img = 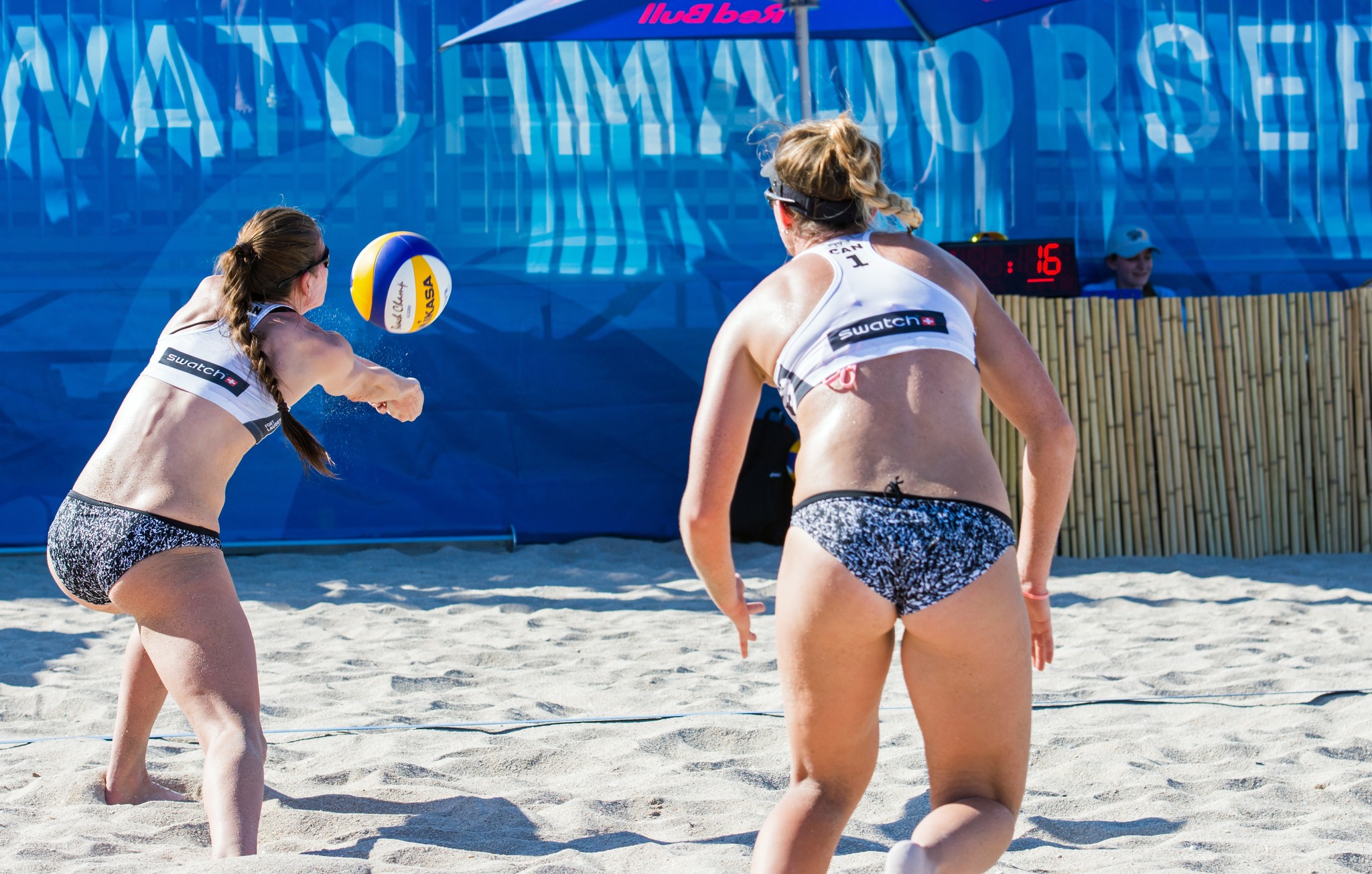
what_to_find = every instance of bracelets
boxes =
[1020,589,1051,603]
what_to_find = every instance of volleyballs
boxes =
[786,439,802,483]
[349,231,452,334]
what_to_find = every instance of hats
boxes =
[1107,225,1160,258]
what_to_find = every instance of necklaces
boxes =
[805,230,841,245]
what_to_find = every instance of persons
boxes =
[1080,224,1176,300]
[45,207,424,859]
[679,111,1077,874]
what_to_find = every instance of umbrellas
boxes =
[441,0,1060,121]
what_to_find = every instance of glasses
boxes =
[277,245,330,289]
[971,232,1008,242]
